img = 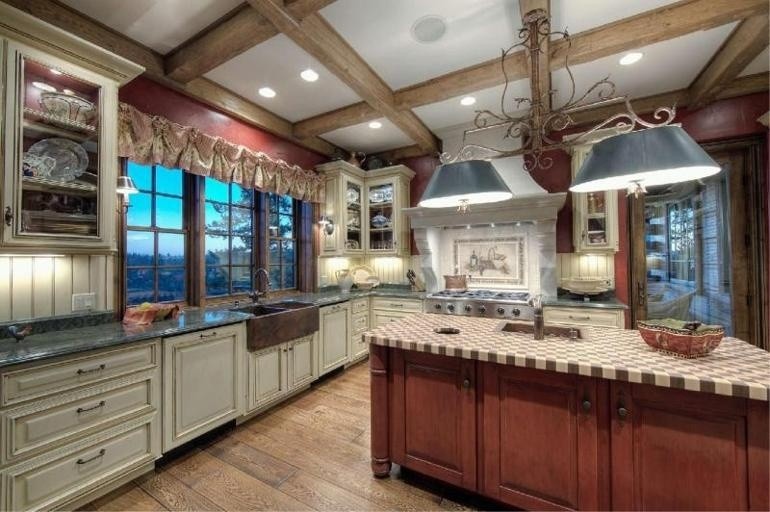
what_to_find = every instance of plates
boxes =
[28,137,90,183]
[353,265,381,290]
[346,186,393,227]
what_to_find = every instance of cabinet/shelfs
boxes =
[344,295,371,370]
[0,336,164,512]
[236,332,319,426]
[390,348,482,495]
[319,300,352,378]
[371,296,425,329]
[315,159,364,257]
[1,2,147,256]
[365,164,416,255]
[163,321,245,456]
[562,125,619,255]
[540,306,625,329]
[482,361,770,512]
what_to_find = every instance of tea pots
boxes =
[21,145,56,179]
[335,269,353,294]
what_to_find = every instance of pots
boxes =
[443,275,467,293]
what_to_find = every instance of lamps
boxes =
[116,176,139,215]
[319,215,334,235]
[417,9,723,214]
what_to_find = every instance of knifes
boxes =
[408,270,415,285]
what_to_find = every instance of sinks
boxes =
[266,302,317,310]
[228,306,287,319]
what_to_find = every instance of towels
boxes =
[122,301,180,326]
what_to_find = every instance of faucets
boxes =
[252,268,273,290]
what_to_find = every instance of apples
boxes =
[141,302,153,310]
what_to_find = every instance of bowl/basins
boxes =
[40,90,97,125]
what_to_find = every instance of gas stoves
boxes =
[423,290,531,318]
